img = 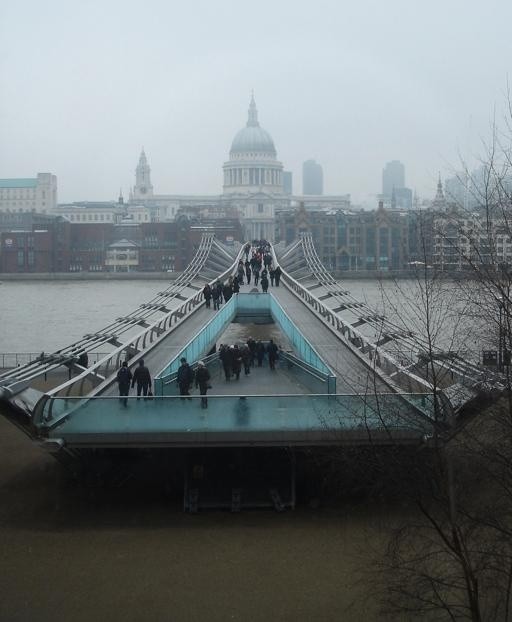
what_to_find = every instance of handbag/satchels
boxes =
[206,383,211,388]
[148,387,153,400]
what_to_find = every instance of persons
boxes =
[195,359,212,409]
[131,359,152,402]
[234,397,249,428]
[202,238,283,311]
[219,335,279,382]
[175,357,194,401]
[117,360,133,408]
